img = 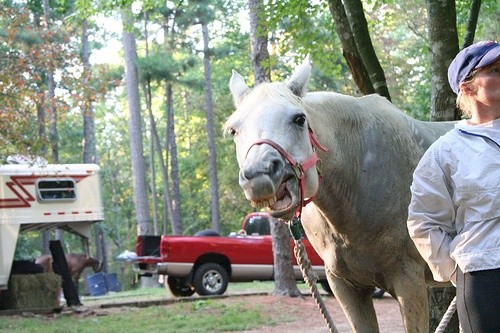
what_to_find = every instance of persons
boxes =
[407,40,500,333]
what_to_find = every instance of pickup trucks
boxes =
[135,212,386,298]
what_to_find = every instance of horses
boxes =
[220,52,468,333]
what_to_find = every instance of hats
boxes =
[448,41,500,95]
